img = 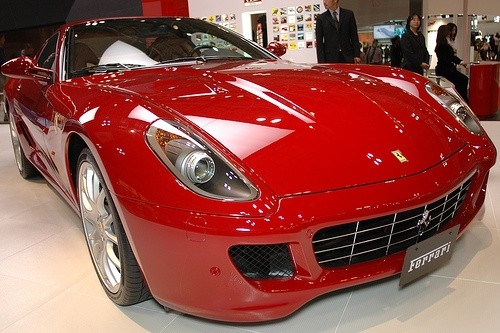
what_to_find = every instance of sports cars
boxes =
[0,15,497,324]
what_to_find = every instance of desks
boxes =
[469,61,500,121]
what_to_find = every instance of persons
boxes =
[0,32,7,65]
[435,25,469,104]
[364,35,400,66]
[313,0,362,65]
[471,31,500,62]
[398,13,430,74]
[446,23,458,69]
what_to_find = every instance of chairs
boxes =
[74,34,199,69]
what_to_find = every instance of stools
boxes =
[428,75,455,87]
